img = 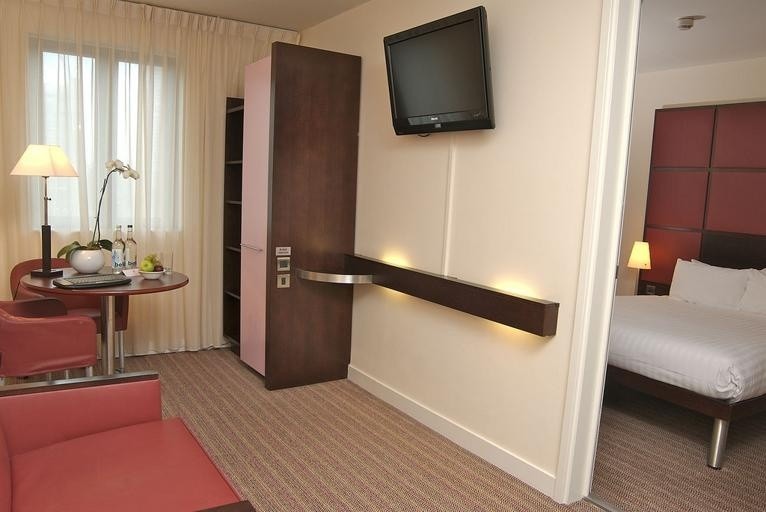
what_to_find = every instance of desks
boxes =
[20,266,190,373]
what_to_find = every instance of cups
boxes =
[160,246,174,276]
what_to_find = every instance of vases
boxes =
[68,245,105,275]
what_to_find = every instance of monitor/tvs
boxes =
[382,5,495,135]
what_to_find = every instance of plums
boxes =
[154,266,163,271]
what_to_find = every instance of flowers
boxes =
[57,158,141,263]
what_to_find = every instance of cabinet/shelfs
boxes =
[241,42,362,390]
[223,96,243,346]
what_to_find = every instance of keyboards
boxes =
[51,273,131,289]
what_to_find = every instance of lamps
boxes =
[9,144,81,277]
[627,240,653,295]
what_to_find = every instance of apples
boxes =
[141,260,154,272]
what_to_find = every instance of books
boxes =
[113,268,143,277]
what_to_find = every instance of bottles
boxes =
[125,224,137,268]
[111,224,126,274]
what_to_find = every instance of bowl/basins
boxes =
[138,269,165,280]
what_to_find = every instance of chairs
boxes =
[10,253,130,369]
[0,297,96,376]
[0,368,259,512]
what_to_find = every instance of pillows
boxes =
[669,258,750,310]
[760,265,765,273]
[691,257,754,271]
[738,268,766,314]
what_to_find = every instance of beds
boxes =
[605,228,766,470]
